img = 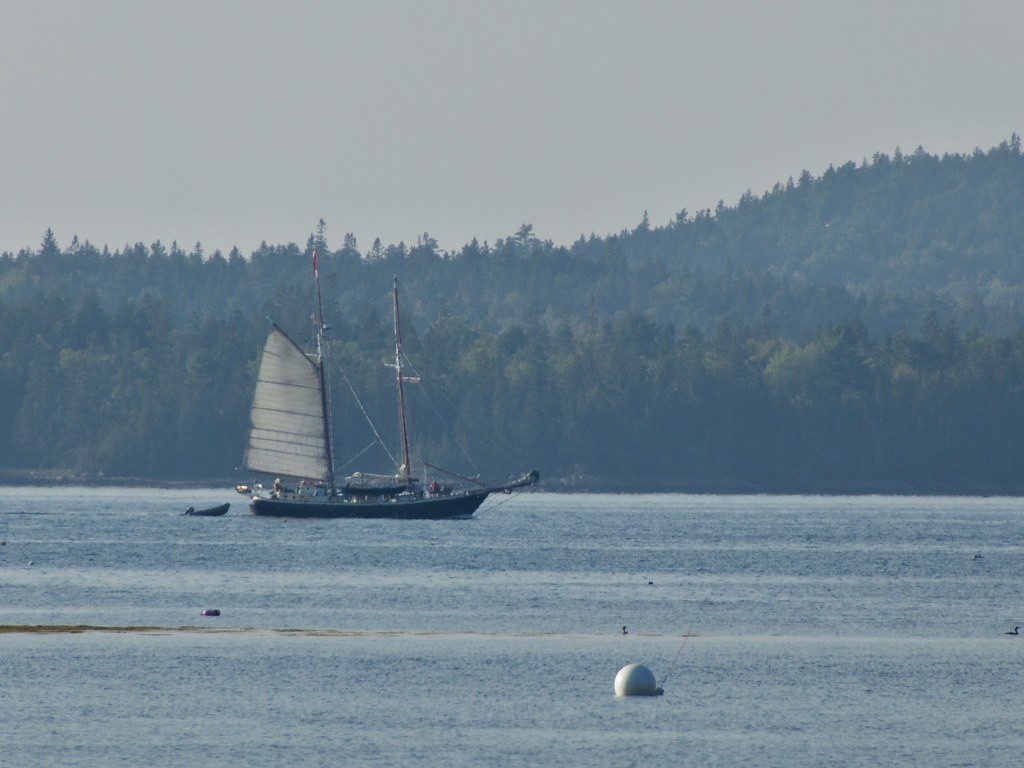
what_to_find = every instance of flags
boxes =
[312,250,317,278]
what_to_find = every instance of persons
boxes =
[274,477,280,498]
[432,481,437,498]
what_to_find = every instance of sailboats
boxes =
[235,249,539,519]
